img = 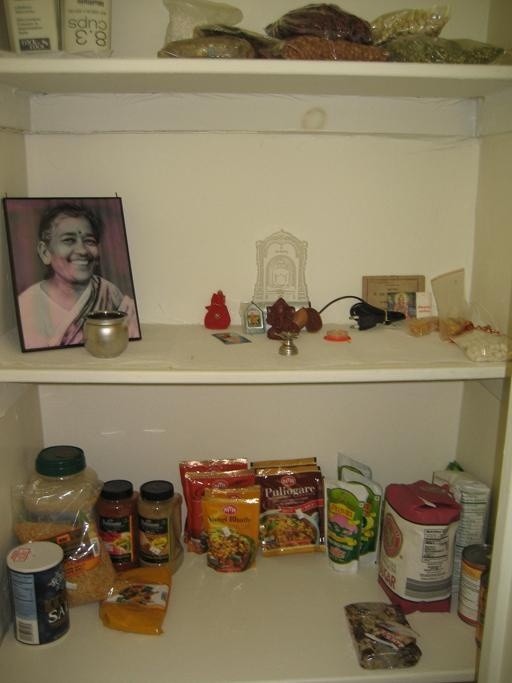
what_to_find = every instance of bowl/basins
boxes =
[83,312,131,360]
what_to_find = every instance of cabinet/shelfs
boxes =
[0,0,511,677]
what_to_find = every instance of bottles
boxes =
[377,481,463,614]
[474,569,489,647]
[136,478,186,574]
[95,478,139,571]
[456,542,492,625]
[5,539,72,646]
[22,444,100,522]
